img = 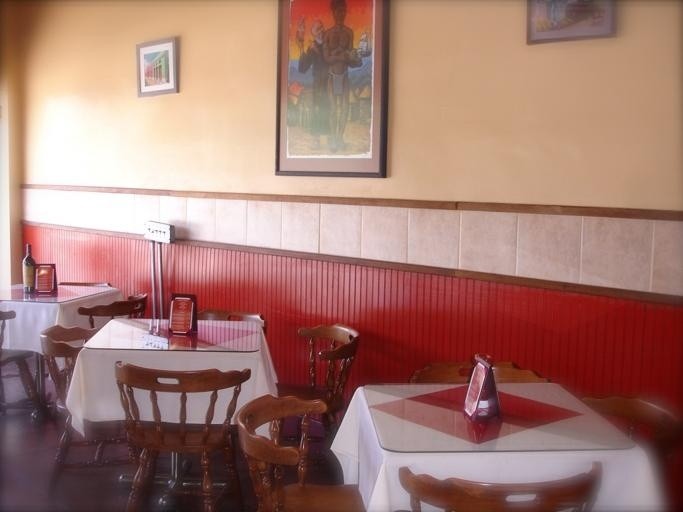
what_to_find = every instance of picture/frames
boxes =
[275,0,388,179]
[136,34,179,98]
[525,0,616,43]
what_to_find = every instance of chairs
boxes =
[1,270,680,512]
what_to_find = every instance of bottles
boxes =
[22,242,35,293]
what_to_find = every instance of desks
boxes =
[361,383,642,512]
[81,317,262,512]
[1,285,120,423]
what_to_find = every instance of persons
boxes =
[321,0,362,155]
[295,15,329,152]
